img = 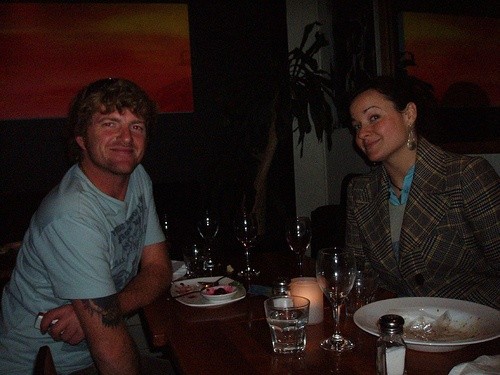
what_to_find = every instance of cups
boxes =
[290,277,324,325]
[345,271,380,316]
[272,278,291,297]
[263,296,310,354]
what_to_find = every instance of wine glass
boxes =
[197,210,220,270]
[160,215,174,260]
[284,216,312,277]
[232,212,262,281]
[315,247,356,351]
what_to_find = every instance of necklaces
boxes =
[382,169,409,193]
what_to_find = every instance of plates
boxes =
[170,275,246,309]
[352,297,500,353]
[201,285,237,299]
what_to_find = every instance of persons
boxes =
[0,76,173,374]
[341,77,500,314]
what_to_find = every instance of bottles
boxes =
[375,314,407,375]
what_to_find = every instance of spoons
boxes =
[210,275,227,286]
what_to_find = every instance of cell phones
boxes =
[34,314,65,335]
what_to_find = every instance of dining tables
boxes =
[140,252,500,375]
